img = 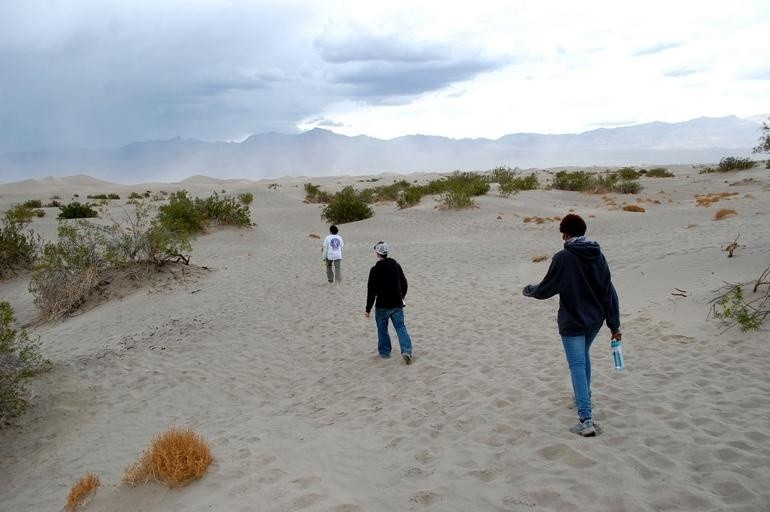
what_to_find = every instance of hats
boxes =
[373,241,389,255]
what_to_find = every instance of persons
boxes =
[322,224,344,284]
[522,213,623,437]
[365,240,413,365]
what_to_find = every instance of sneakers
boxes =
[401,352,413,365]
[574,420,596,437]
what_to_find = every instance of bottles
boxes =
[609,338,625,371]
